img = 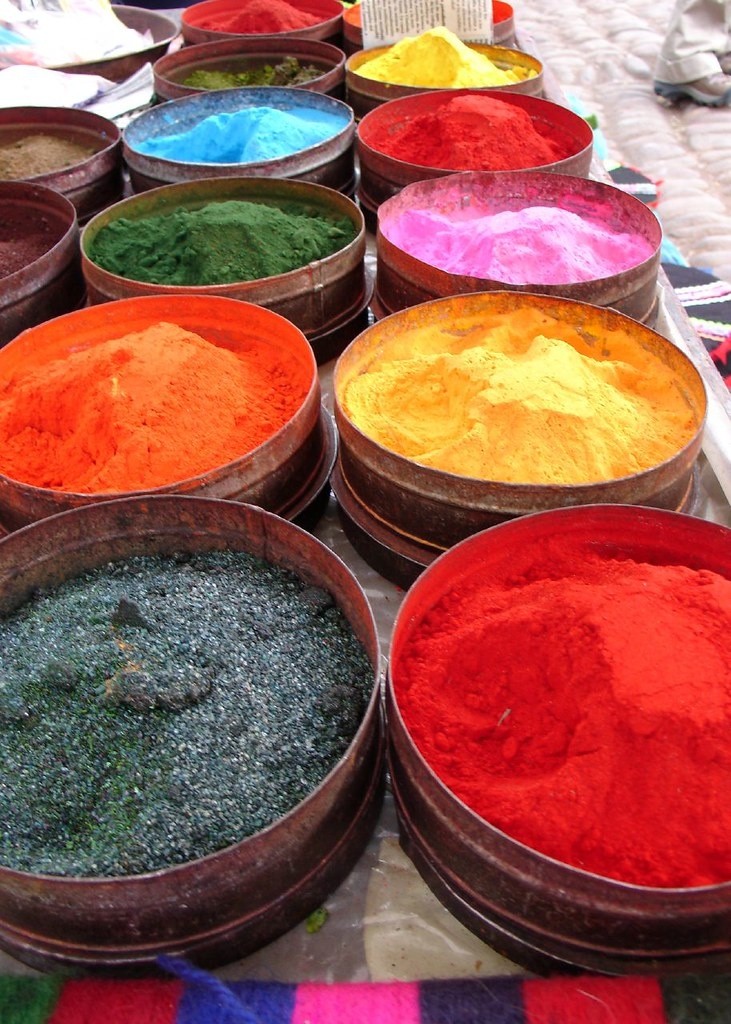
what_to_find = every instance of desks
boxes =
[0,0,731,1024]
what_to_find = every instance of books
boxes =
[84,64,155,118]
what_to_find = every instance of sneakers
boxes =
[653,71,731,107]
[719,55,731,74]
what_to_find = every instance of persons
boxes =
[653,0,731,107]
